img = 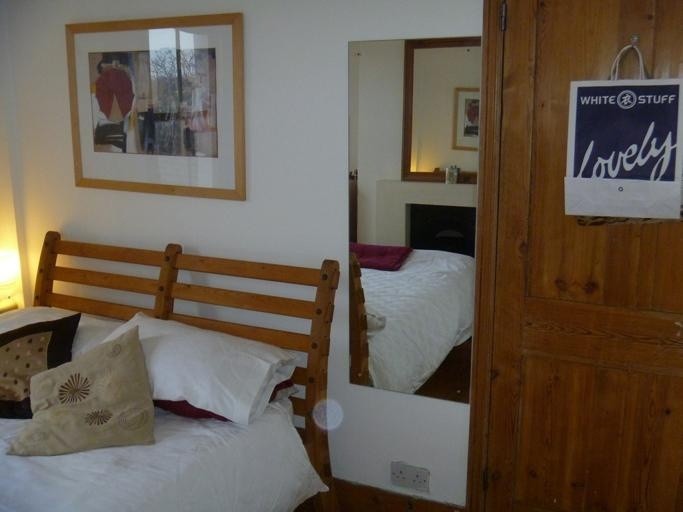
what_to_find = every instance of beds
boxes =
[349,228,474,395]
[1,232,339,511]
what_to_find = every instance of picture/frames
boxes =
[402,38,482,184]
[65,11,248,203]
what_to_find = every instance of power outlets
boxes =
[390,461,430,492]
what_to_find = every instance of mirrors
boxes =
[347,35,487,411]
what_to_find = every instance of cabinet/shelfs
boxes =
[461,0,683,512]
